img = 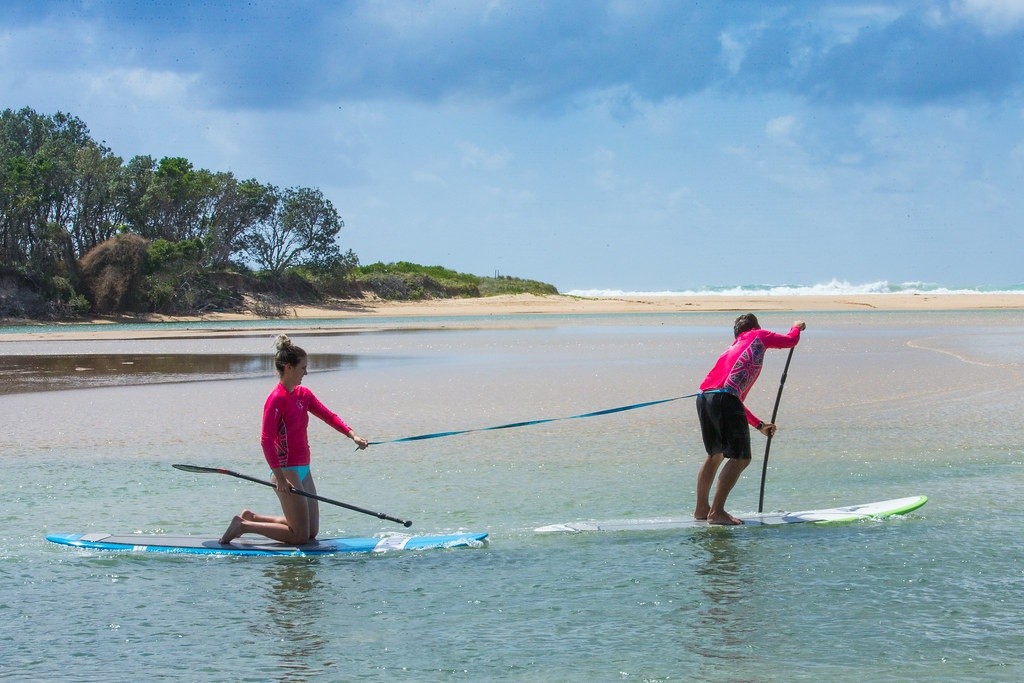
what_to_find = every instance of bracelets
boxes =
[757,422,763,429]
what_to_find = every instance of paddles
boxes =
[758,321,807,514]
[171,463,413,528]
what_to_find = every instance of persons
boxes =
[217,334,369,544]
[695,312,807,525]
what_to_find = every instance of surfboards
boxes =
[532,495,929,533]
[45,532,489,557]
